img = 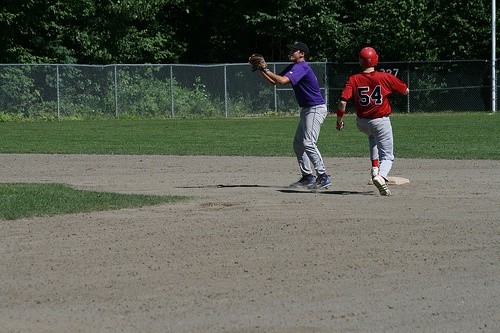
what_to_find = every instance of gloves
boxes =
[336,121,345,131]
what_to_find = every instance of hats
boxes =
[287,42,309,57]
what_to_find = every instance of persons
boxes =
[249,41,332,190]
[336,47,409,196]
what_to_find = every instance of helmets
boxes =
[359,47,378,67]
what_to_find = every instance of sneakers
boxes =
[372,175,391,196]
[307,173,332,190]
[368,176,375,185]
[290,174,317,188]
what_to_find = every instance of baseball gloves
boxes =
[249,54,266,70]
